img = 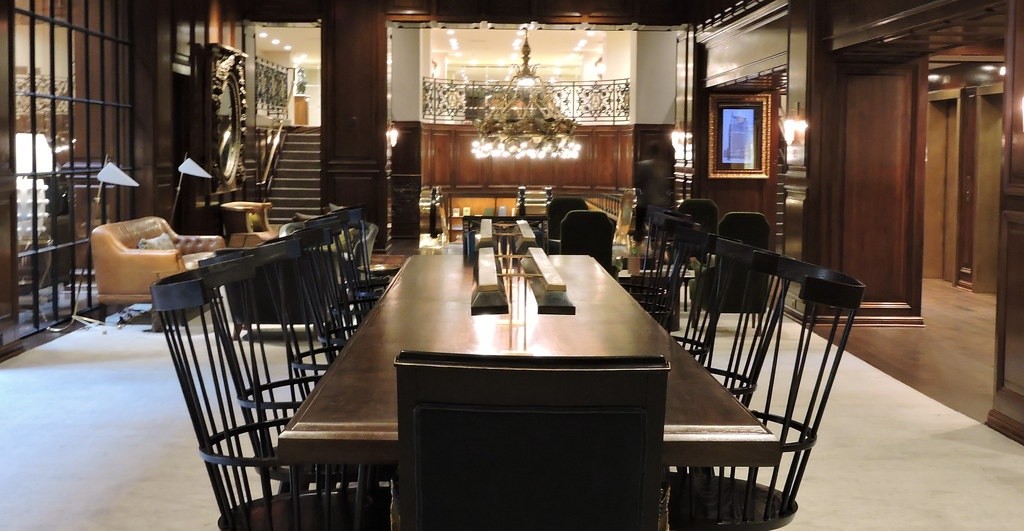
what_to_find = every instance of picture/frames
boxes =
[707,93,771,180]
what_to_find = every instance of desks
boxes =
[275,255,783,531]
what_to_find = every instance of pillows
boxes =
[137,232,175,250]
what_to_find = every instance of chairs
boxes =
[617,197,867,531]
[394,352,672,531]
[148,209,401,531]
[547,197,588,255]
[561,210,615,274]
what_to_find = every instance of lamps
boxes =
[470,28,582,161]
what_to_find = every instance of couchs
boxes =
[91,217,225,325]
[220,201,273,239]
